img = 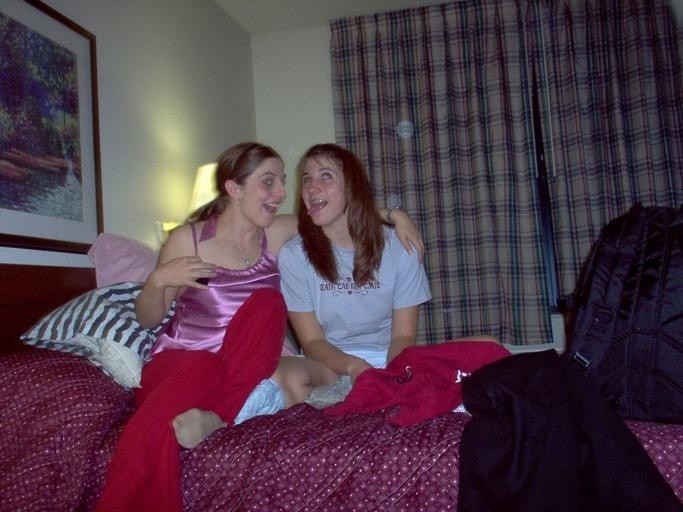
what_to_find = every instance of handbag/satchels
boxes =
[323,342,512,427]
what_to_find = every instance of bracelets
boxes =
[385,205,402,225]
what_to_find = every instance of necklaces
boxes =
[334,247,351,283]
[218,215,256,266]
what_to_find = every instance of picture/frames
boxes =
[0,0,104,255]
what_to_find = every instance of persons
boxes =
[268,145,504,410]
[99,139,429,512]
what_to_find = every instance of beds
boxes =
[1,262,681,511]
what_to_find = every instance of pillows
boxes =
[17,231,177,392]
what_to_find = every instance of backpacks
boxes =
[562,201,683,424]
